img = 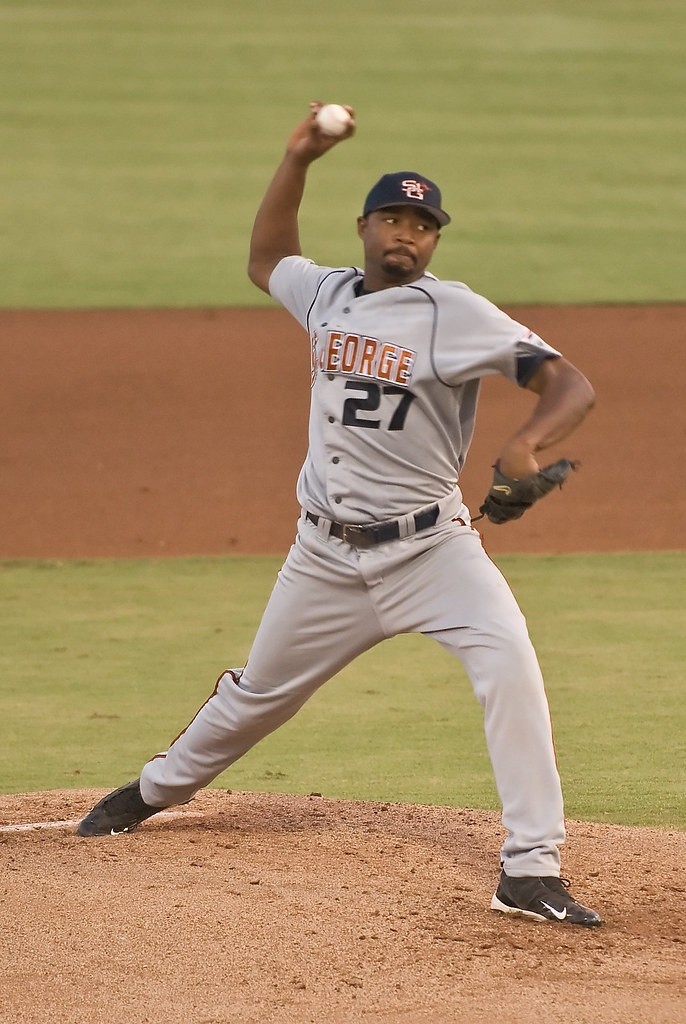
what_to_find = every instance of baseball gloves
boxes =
[471,456,582,526]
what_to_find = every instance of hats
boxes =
[363,171,451,226]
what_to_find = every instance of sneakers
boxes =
[489,861,600,927]
[75,777,195,837]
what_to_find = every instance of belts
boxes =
[307,507,439,546]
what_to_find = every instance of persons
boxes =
[77,97,603,929]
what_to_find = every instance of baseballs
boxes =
[315,103,351,136]
